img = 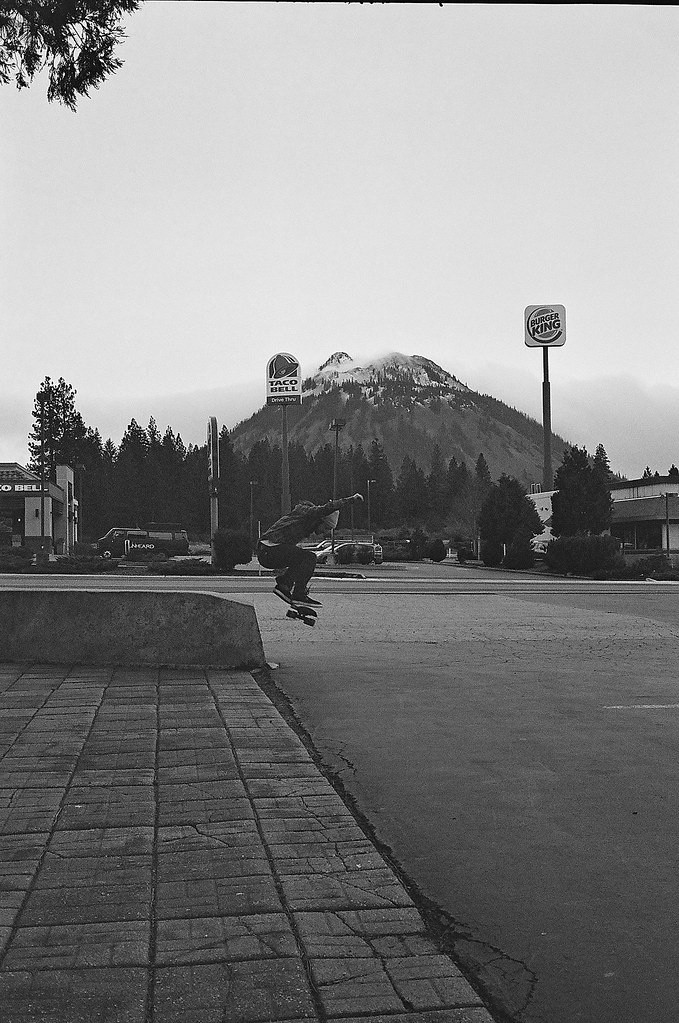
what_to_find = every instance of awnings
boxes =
[544,498,667,527]
[668,497,679,524]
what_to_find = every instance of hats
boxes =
[322,509,339,529]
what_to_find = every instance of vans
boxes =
[93,527,190,558]
[302,539,384,566]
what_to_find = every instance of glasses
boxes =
[323,522,332,531]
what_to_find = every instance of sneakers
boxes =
[292,584,324,607]
[273,583,292,605]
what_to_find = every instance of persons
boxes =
[257,493,364,607]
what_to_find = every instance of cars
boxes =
[530,542,549,561]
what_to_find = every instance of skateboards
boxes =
[285,604,317,627]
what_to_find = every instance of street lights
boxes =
[35,391,53,562]
[367,479,377,535]
[249,480,260,557]
[328,418,347,555]
